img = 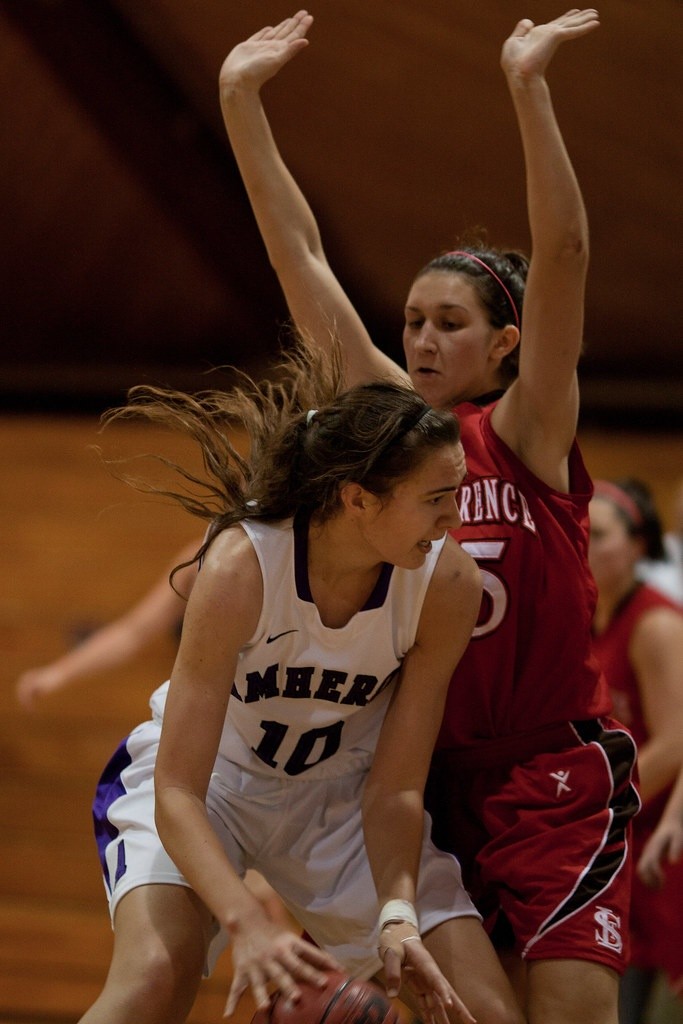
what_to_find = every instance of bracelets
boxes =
[371,898,420,949]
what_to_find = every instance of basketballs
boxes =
[246,970,407,1024]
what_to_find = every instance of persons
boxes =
[591,477,683,1024]
[17,532,205,706]
[218,8,642,1024]
[78,315,526,1024]
